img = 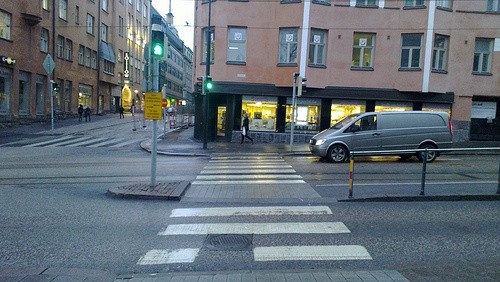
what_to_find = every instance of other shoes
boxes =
[240,142,244,144]
[252,139,254,144]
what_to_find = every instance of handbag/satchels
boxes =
[242,127,246,136]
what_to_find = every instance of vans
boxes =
[308,111,454,163]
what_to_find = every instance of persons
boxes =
[240,113,254,144]
[78,104,83,121]
[221,120,225,129]
[111,103,114,112]
[120,105,124,118]
[131,104,143,130]
[84,106,91,122]
[367,116,376,129]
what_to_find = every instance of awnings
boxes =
[101,42,116,64]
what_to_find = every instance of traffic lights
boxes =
[151,30,164,57]
[205,76,212,91]
[298,76,307,98]
[196,76,205,95]
